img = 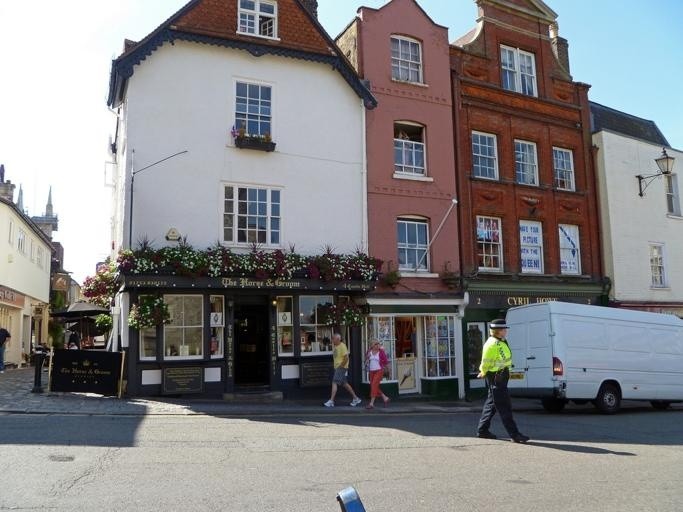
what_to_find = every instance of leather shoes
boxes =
[477,431,496,439]
[511,432,529,443]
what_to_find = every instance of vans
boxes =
[503,301,683,415]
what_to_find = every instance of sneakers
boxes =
[349,397,361,407]
[323,400,335,407]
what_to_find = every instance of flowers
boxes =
[231,126,247,141]
[82,240,383,329]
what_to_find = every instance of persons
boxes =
[0,328,11,373]
[478,319,529,443]
[324,333,361,407]
[366,339,389,408]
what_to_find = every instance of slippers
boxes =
[365,404,374,409]
[383,398,389,408]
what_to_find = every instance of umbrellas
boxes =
[50,300,111,350]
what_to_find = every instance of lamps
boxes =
[636,146,676,196]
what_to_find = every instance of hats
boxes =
[489,319,510,330]
